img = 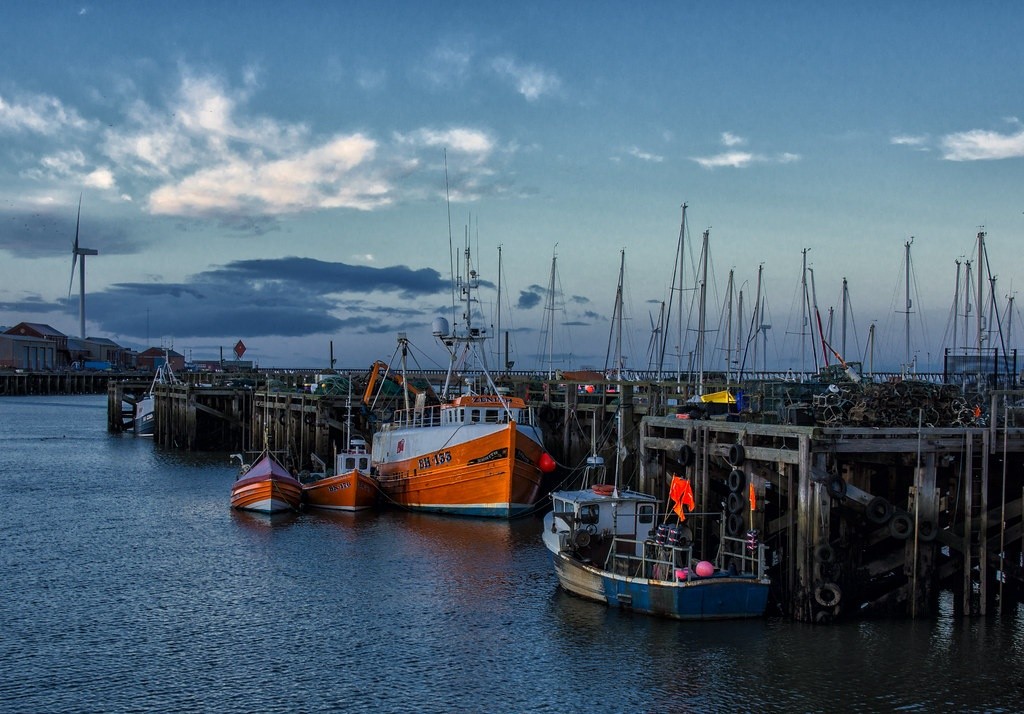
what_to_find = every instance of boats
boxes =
[368,145,544,519]
[231,381,302,514]
[134,337,189,437]
[297,374,381,511]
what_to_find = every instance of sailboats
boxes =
[540,248,772,621]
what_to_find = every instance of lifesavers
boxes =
[543,408,556,422]
[729,444,745,466]
[889,512,914,539]
[727,493,744,513]
[918,518,938,542]
[816,611,833,624]
[592,484,621,496]
[728,514,743,535]
[304,414,314,424]
[278,414,285,425]
[866,496,892,524]
[680,444,693,466]
[826,474,847,499]
[728,470,746,493]
[814,544,841,582]
[814,583,841,607]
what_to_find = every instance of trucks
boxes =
[71,361,112,371]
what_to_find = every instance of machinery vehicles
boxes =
[362,359,426,420]
